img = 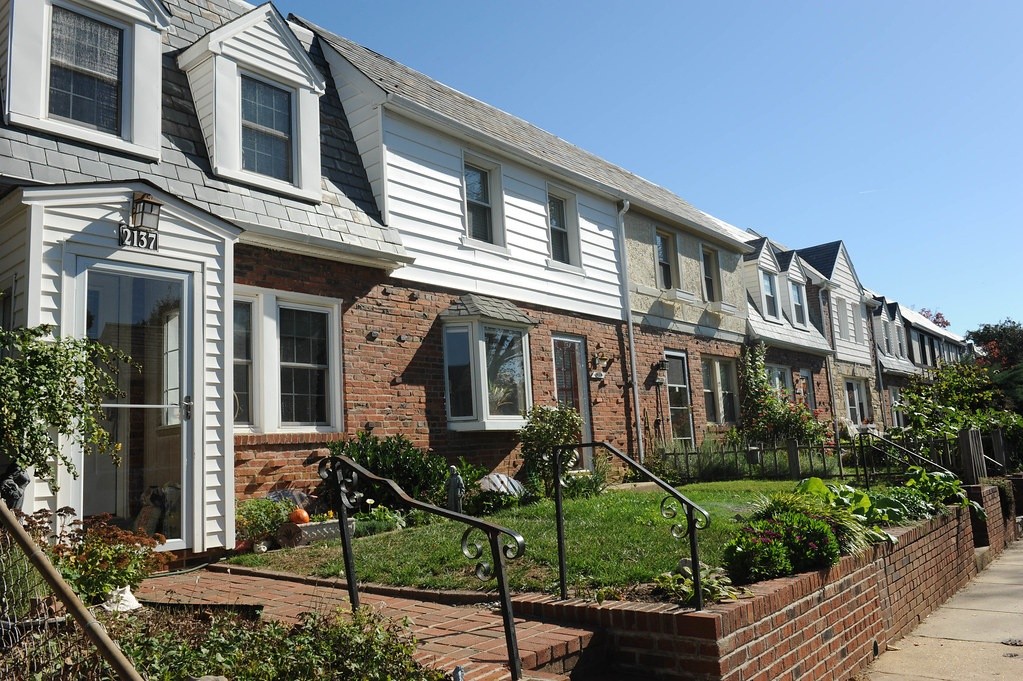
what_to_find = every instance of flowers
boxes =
[309,510,334,521]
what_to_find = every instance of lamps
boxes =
[132,192,164,231]
[594,351,608,367]
[652,359,670,370]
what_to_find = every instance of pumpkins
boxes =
[290,505,309,524]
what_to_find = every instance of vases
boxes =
[277,518,358,548]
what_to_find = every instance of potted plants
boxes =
[235,497,296,552]
[861,415,877,428]
